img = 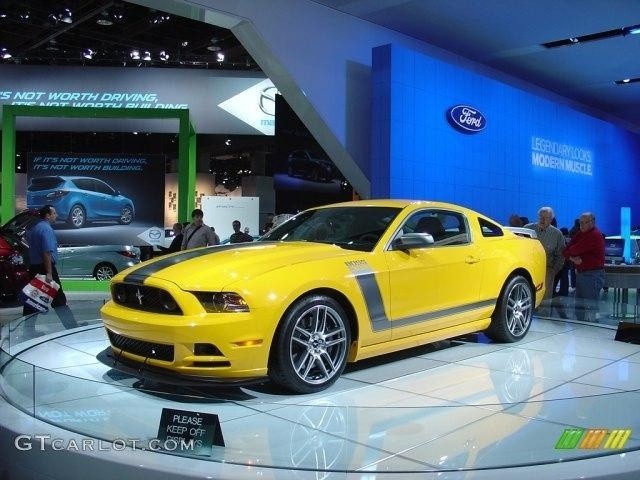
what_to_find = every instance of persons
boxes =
[21,204,89,339]
[506,205,606,325]
[156,209,253,254]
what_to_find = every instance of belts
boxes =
[576,268,602,273]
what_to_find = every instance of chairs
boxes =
[413,218,444,242]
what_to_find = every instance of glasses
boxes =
[580,219,591,224]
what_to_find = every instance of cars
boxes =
[100,198,548,395]
[0,209,43,303]
[288,149,334,182]
[27,176,135,227]
[55,243,141,280]
[207,147,253,190]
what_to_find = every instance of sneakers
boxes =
[23,333,45,340]
[76,322,88,327]
